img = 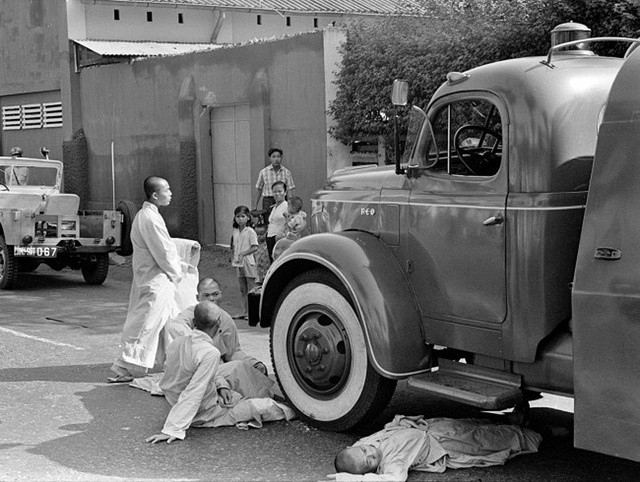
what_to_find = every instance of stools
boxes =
[251,209,269,231]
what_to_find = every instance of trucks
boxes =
[248,22,640,463]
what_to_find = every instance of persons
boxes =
[278,197,304,239]
[169,277,267,386]
[266,182,287,266]
[145,301,300,444]
[254,148,296,235]
[327,412,572,482]
[231,206,259,320]
[105,176,200,381]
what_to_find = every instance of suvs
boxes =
[0,147,136,288]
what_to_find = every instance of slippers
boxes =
[107,374,134,382]
